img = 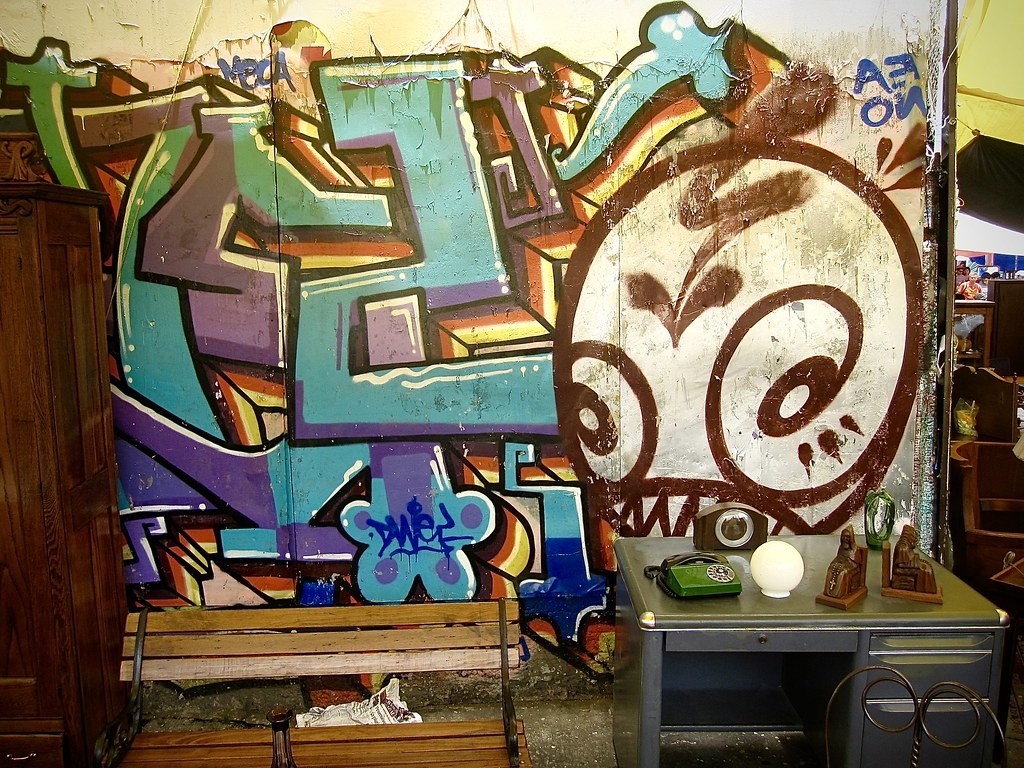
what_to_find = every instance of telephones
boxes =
[662,551,742,600]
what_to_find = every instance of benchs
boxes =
[89,597,535,768]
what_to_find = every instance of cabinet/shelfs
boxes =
[612,532,1012,767]
[0,182,132,767]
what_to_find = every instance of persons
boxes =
[821,524,861,600]
[893,523,940,595]
[955,260,1024,302]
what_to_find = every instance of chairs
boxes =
[950,439,1024,600]
